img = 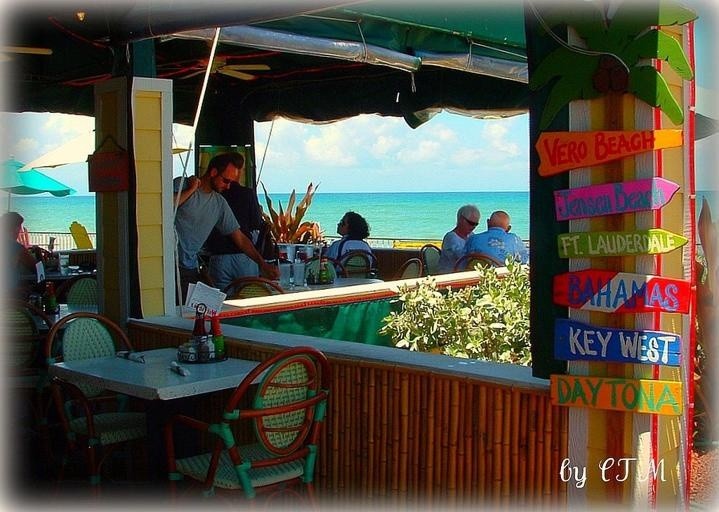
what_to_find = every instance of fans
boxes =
[0,46,53,61]
[179,52,271,81]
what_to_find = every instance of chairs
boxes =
[0,244,507,512]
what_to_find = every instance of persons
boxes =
[0,211,39,292]
[172,153,280,304]
[459,211,528,272]
[321,211,374,279]
[202,154,263,299]
[432,206,480,276]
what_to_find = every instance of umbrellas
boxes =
[0,154,79,212]
[15,131,192,173]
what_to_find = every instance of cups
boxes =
[276,262,307,288]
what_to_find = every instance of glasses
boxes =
[463,216,478,226]
[219,174,232,185]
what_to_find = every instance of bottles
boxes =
[27,281,61,316]
[304,246,338,287]
[178,310,225,362]
